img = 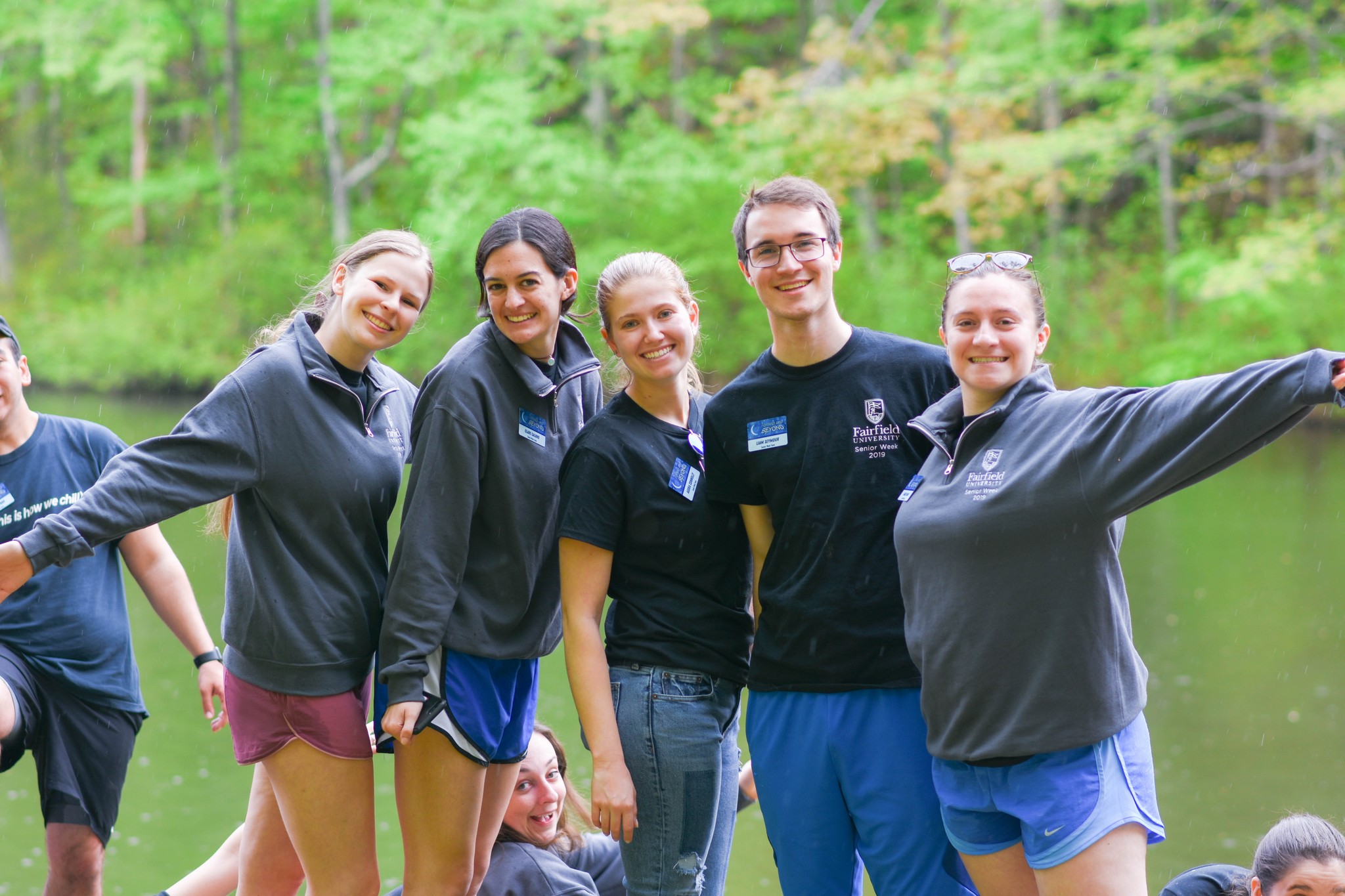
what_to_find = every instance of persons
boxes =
[559,252,752,896]
[0,227,431,896]
[893,251,1345,896]
[381,719,760,896]
[372,206,602,896]
[703,176,980,896]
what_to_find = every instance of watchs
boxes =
[193,646,223,668]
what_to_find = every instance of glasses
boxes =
[744,238,829,268]
[945,251,1043,302]
